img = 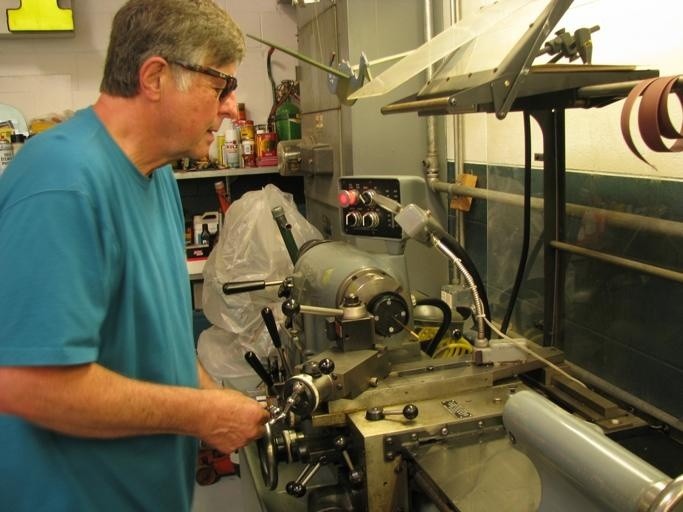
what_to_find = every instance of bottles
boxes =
[200,223,213,257]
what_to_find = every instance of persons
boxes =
[0,1,273,509]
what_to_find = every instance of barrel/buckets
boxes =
[194,211,218,244]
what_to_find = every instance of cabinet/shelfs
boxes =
[293,1,456,309]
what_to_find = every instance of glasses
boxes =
[163,56,237,104]
